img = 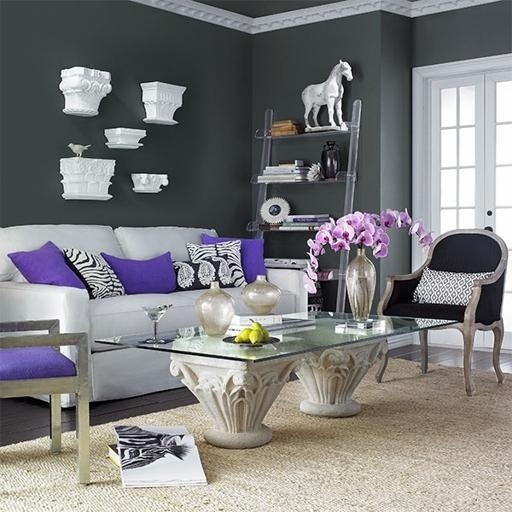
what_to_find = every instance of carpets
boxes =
[0,356,512,512]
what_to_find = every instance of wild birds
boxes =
[67,143,91,157]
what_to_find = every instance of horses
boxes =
[301,59,353,129]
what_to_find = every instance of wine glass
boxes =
[138,304,174,344]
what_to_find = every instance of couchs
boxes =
[0,224,309,411]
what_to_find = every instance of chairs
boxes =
[0,319,90,484]
[376,229,508,396]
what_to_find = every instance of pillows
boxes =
[411,266,495,307]
[7,233,268,299]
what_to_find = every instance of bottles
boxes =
[319,139,344,180]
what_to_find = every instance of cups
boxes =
[175,326,196,341]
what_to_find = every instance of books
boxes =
[113,424,208,487]
[257,120,329,231]
[228,318,315,332]
[269,325,316,335]
[107,444,120,468]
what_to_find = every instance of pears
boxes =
[233,322,269,345]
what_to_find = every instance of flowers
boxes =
[303,209,433,317]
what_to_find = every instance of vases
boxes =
[346,247,376,322]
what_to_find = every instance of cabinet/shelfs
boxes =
[246,99,362,317]
